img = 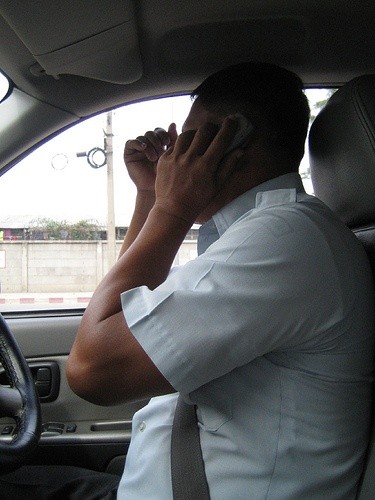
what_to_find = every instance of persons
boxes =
[0,64,374,500]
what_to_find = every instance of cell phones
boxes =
[197,114,256,158]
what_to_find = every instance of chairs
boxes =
[308,69,375,500]
